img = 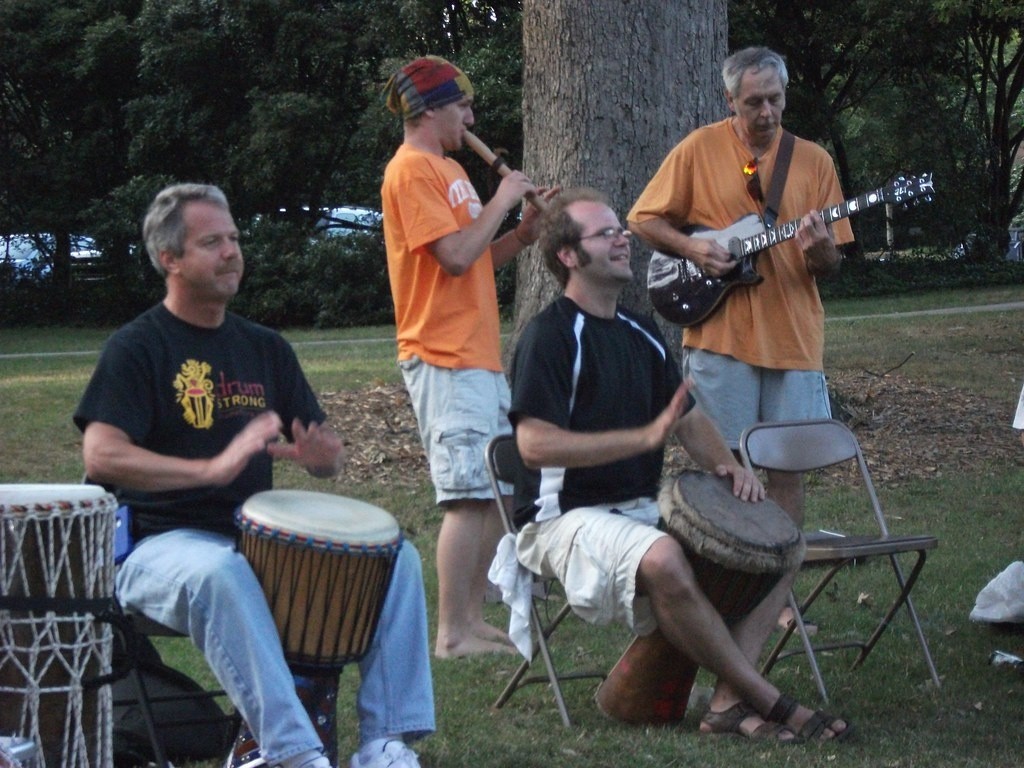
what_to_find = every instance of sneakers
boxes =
[350,747,423,768]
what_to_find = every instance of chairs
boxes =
[739,422,942,705]
[82,471,244,768]
[489,435,608,729]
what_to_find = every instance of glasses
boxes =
[744,158,764,203]
[580,227,634,238]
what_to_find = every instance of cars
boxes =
[954,230,1022,263]
[0,232,137,279]
[245,205,384,247]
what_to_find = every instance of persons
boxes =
[506,191,854,744]
[73,184,436,768]
[627,47,856,637]
[381,56,561,659]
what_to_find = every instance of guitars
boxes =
[646,169,939,330]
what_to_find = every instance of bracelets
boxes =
[515,227,531,245]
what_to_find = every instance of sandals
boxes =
[698,702,806,747]
[763,693,854,745]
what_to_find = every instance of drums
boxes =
[227,487,406,672]
[589,466,808,730]
[1,480,121,768]
